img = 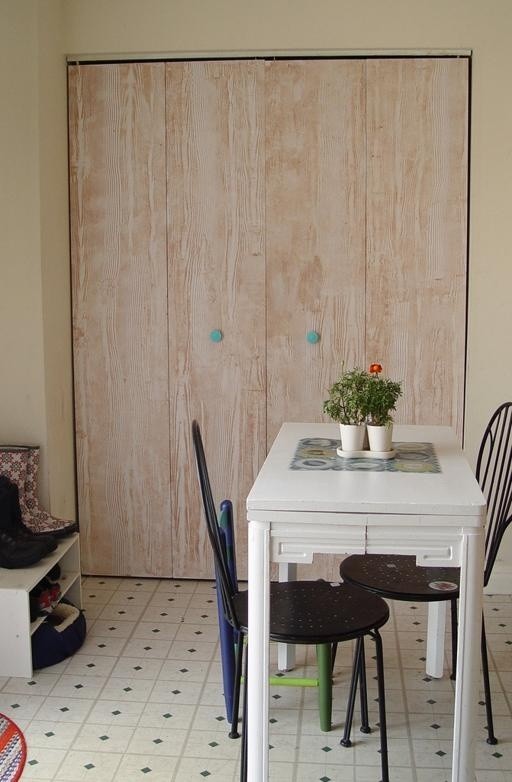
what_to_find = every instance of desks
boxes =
[242,421,489,782]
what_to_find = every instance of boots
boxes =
[0,445,79,569]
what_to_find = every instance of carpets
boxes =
[0,710,30,782]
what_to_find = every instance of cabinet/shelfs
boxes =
[1,530,88,680]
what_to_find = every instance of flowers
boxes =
[321,363,404,424]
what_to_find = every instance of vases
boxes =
[337,423,395,460]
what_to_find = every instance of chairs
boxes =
[209,500,336,733]
[190,419,390,781]
[339,400,512,749]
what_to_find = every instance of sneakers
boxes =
[29,583,61,622]
